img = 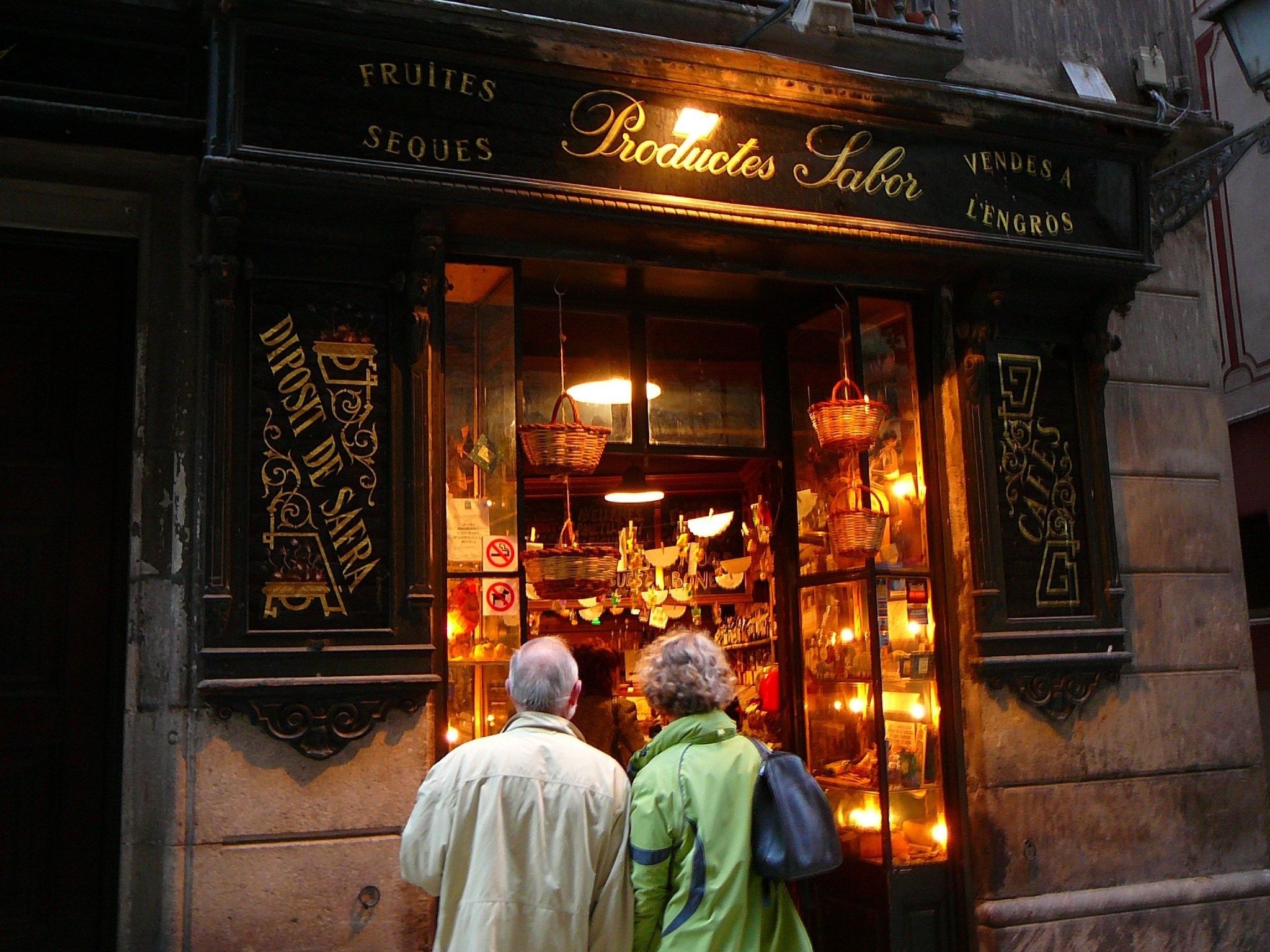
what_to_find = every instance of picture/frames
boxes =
[843,312,912,399]
[645,359,767,449]
[521,357,633,444]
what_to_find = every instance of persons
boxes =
[569,639,649,772]
[628,632,816,952]
[399,638,635,952]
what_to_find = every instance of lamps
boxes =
[565,347,663,404]
[604,461,665,503]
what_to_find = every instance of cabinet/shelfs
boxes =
[449,472,969,952]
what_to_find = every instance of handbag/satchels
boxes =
[748,737,843,882]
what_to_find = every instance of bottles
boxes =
[714,606,777,645]
